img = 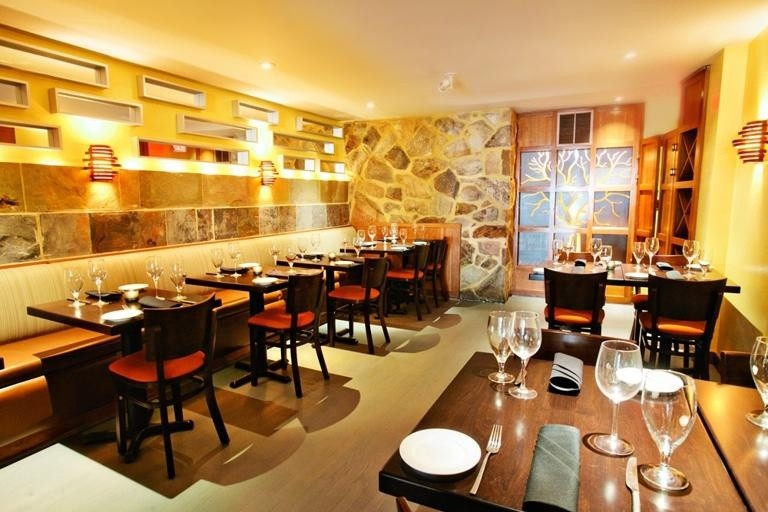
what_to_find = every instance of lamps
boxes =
[259,161,277,186]
[83,145,122,182]
[733,120,768,164]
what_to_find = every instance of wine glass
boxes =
[583,337,642,458]
[744,334,768,431]
[634,365,694,494]
[145,256,165,301]
[87,259,110,306]
[269,231,321,267]
[227,242,242,276]
[340,221,408,258]
[65,266,86,307]
[551,236,711,278]
[211,247,225,278]
[505,309,543,403]
[483,308,517,387]
[167,263,187,300]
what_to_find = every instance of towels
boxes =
[523,423,581,512]
[656,262,673,270]
[550,352,584,393]
[575,259,586,266]
[85,290,121,301]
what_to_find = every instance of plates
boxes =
[395,428,481,477]
[251,277,277,285]
[412,239,427,246]
[615,363,684,392]
[119,283,148,290]
[239,262,260,267]
[101,308,140,324]
[335,261,353,265]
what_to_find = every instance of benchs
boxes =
[0,225,362,469]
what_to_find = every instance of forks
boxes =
[468,422,505,497]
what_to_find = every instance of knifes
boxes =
[623,452,641,511]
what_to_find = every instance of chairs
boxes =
[542,267,608,337]
[385,244,431,321]
[109,293,218,479]
[720,351,768,388]
[629,254,690,342]
[559,252,599,262]
[514,325,637,366]
[247,265,325,398]
[638,274,727,380]
[405,236,448,308]
[329,256,391,352]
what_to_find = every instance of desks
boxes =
[697,378,768,512]
